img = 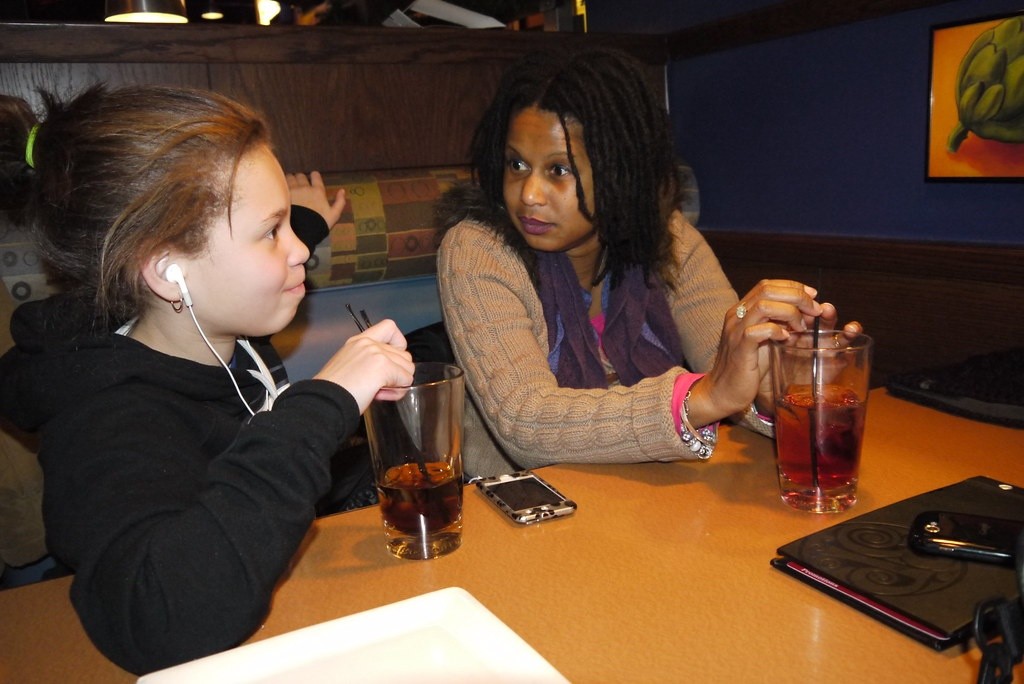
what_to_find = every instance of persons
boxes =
[434,43,865,483]
[0,82,416,677]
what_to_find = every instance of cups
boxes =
[364,361,467,563]
[769,325,874,514]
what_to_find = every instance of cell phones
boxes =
[476,471,578,525]
[908,510,1024,569]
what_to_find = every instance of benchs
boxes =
[0,163,701,588]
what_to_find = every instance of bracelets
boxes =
[751,402,775,429]
[680,391,718,459]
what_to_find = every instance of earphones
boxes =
[166,263,192,307]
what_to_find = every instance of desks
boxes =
[0,390,1024,684]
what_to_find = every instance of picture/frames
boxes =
[924,11,1023,185]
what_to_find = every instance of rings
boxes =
[833,333,842,348]
[736,302,748,319]
